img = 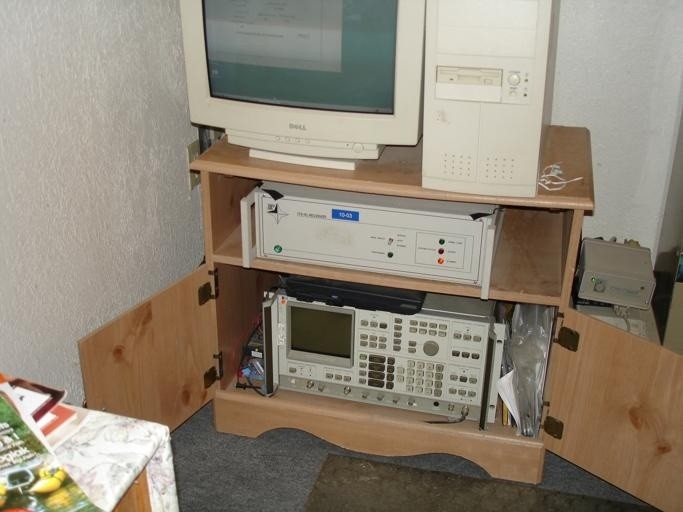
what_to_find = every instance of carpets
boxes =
[305,454,657,511]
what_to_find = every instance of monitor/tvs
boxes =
[174,0,426,159]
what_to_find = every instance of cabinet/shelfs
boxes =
[0,403,178,510]
[76,127,683,512]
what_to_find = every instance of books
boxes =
[0,374,104,510]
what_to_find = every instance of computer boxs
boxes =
[421,0,555,196]
